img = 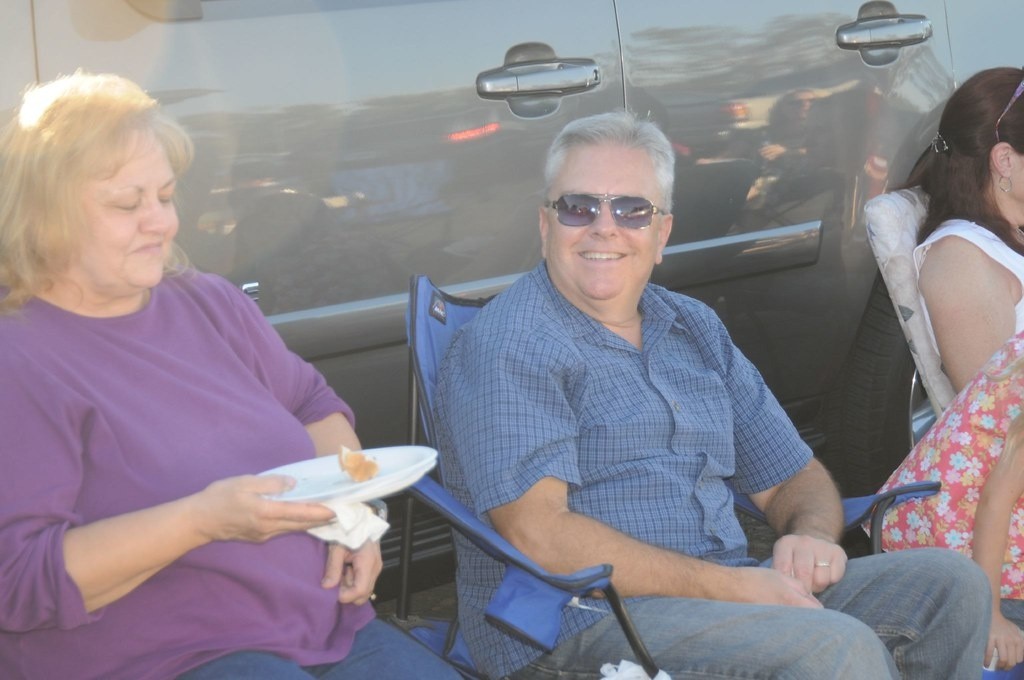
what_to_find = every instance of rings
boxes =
[368,592,376,600]
[814,561,830,567]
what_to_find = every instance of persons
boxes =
[840,68,1024,680]
[433,110,992,680]
[744,87,837,205]
[0,66,466,680]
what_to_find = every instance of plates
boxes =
[254,445,438,508]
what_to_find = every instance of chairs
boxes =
[386,272,941,680]
[861,186,959,453]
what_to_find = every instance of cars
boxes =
[1,1,1024,629]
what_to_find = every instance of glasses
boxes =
[546,194,666,229]
